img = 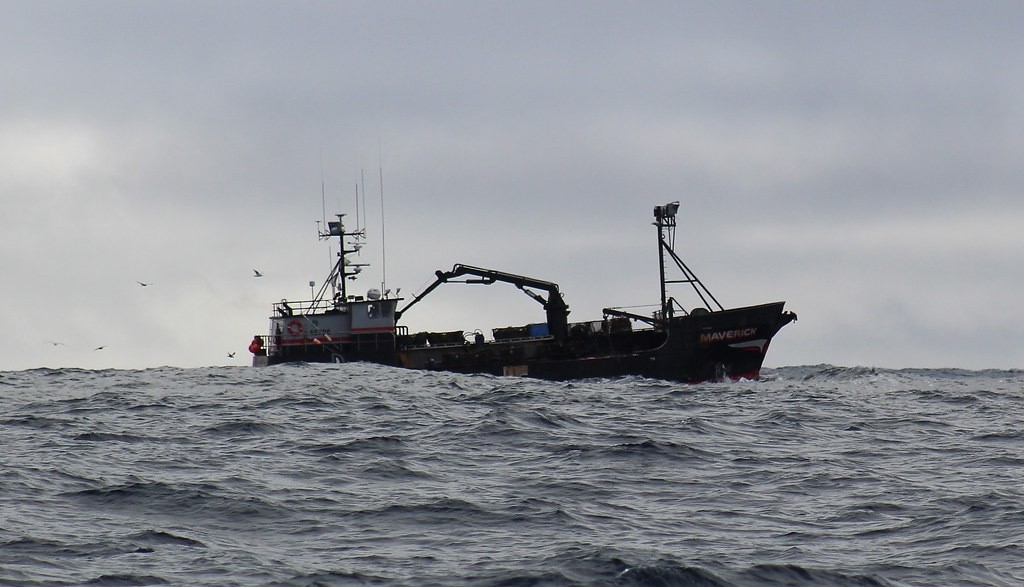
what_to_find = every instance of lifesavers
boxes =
[287,320,304,335]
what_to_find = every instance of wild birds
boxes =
[137,281,152,287]
[93,346,105,352]
[47,341,65,346]
[253,270,263,278]
[227,352,236,358]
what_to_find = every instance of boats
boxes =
[248,168,405,367]
[394,200,799,384]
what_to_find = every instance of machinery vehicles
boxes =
[392,263,570,340]
